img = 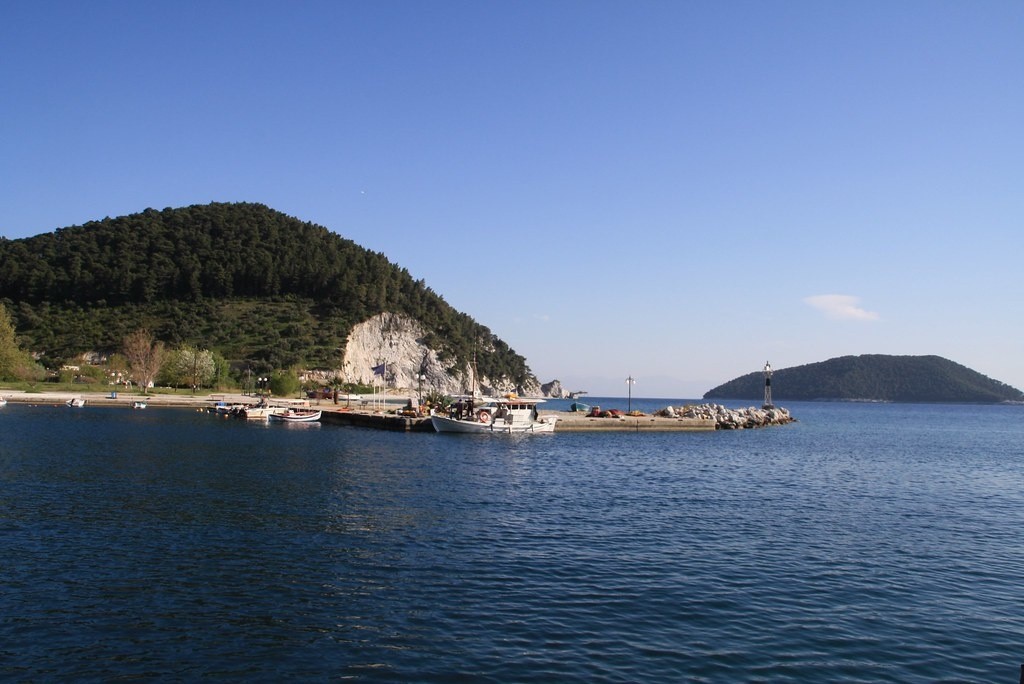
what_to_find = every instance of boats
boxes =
[206,398,322,422]
[431,415,558,432]
[569,392,579,400]
[306,390,360,399]
[130,402,147,409]
[65,399,87,407]
[570,402,590,411]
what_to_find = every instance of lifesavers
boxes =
[480,412,489,422]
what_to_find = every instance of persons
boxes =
[407,398,412,407]
[334,389,338,405]
[455,398,463,420]
[258,394,269,409]
[465,397,474,417]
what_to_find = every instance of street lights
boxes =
[258,377,267,395]
[625,376,635,411]
[414,371,426,417]
[299,372,304,399]
[111,371,122,399]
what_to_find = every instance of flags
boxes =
[511,389,516,392]
